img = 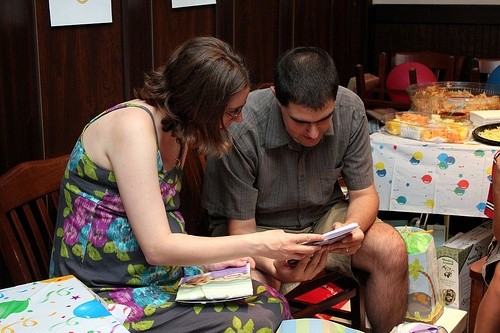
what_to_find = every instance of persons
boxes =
[201,45,410,333]
[50,38,324,333]
[472,157,500,333]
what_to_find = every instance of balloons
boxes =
[386,61,438,104]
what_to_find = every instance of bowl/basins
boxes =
[405,81,500,120]
[380,111,474,144]
[439,111,467,119]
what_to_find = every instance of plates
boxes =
[472,122,500,146]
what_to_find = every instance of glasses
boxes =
[221,106,243,122]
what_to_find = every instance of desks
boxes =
[0,275,131,333]
[370,110,500,241]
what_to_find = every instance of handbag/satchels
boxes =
[394,225,445,325]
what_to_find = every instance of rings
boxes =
[345,247,348,254]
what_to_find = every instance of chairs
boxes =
[355,51,500,110]
[0,154,70,285]
[185,140,365,333]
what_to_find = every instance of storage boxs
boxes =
[436,220,494,333]
